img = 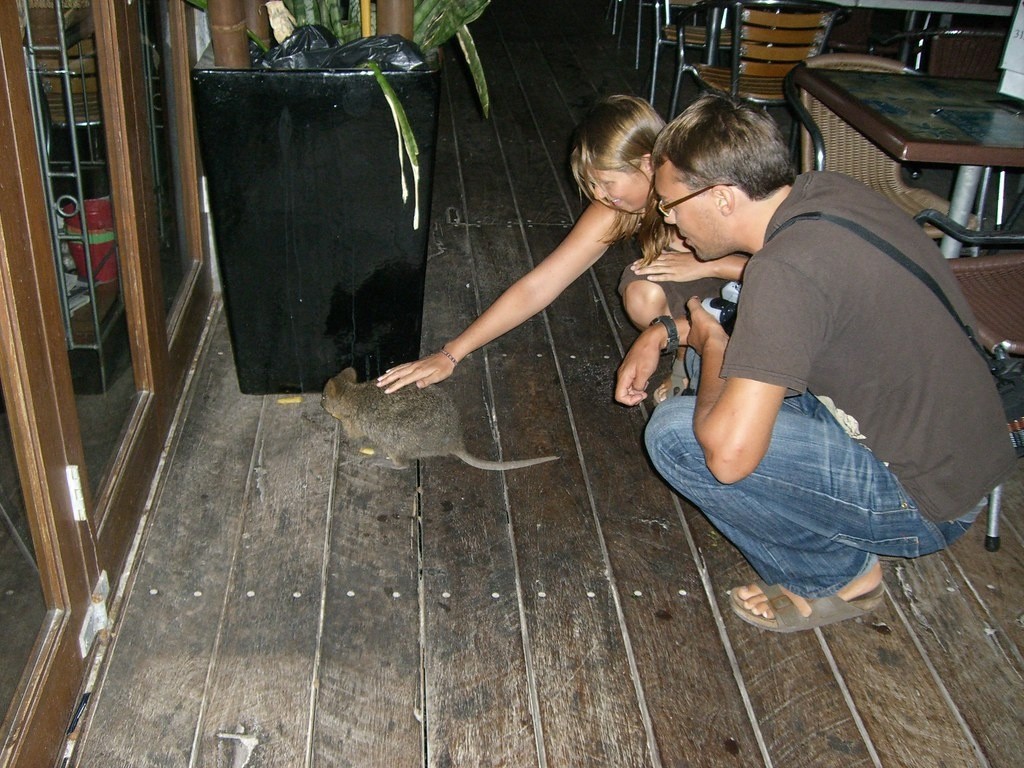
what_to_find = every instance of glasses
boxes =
[658,183,736,217]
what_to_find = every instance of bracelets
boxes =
[439,349,457,366]
[649,316,678,353]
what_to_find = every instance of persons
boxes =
[377,95,751,405]
[615,95,1024,634]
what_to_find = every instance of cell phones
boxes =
[986,99,1024,115]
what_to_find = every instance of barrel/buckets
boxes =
[63,199,117,281]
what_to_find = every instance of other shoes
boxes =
[652,354,690,406]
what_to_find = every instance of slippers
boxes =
[727,577,885,633]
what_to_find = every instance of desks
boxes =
[820,1,1015,68]
[793,67,1024,258]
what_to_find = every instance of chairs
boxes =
[611,0,1024,551]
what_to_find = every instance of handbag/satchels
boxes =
[986,343,1024,458]
